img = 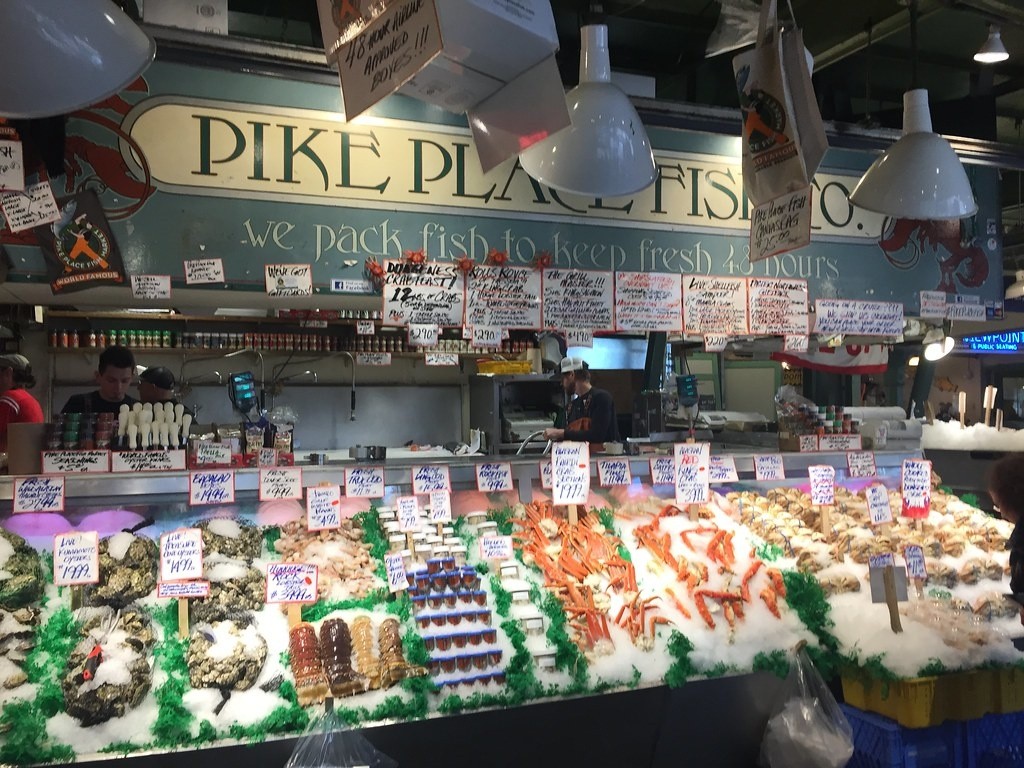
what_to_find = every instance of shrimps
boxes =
[289,614,430,703]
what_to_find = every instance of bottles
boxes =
[51,327,533,353]
[780,400,860,434]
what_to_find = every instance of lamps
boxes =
[0,0,157,118]
[922,324,955,360]
[846,0,978,220]
[1005,258,1024,299]
[519,0,660,199]
[974,22,1009,64]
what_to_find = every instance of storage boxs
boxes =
[837,661,1023,768]
[777,433,864,452]
[315,0,572,175]
[40,421,294,474]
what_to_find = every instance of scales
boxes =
[226,371,258,458]
[657,374,707,449]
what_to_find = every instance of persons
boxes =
[0,353,45,454]
[986,451,1024,629]
[136,365,198,425]
[543,357,620,453]
[60,345,143,421]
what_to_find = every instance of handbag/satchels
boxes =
[283,704,399,768]
[756,649,854,768]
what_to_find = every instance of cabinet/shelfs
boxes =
[47,309,542,358]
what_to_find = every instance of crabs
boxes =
[724,489,1021,648]
[504,495,789,657]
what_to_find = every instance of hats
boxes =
[549,357,588,381]
[0,354,30,373]
[133,365,175,389]
